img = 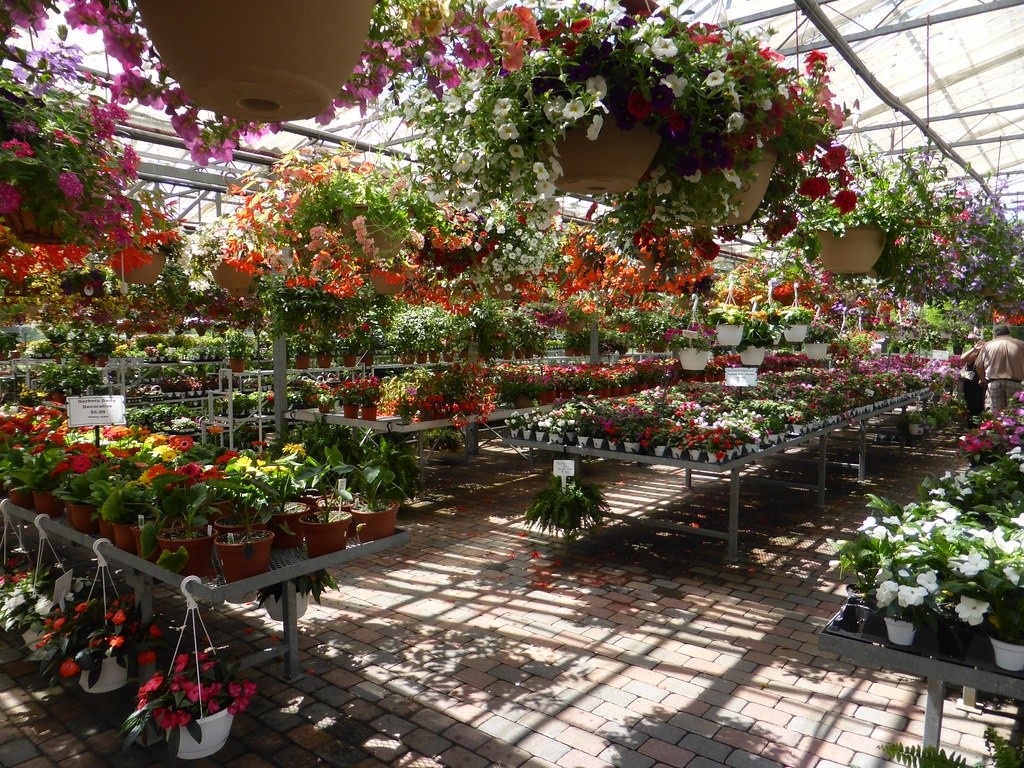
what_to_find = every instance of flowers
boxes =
[0,0,1024,768]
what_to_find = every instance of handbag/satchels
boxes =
[960,361,975,381]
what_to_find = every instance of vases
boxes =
[299,511,352,556]
[536,111,662,193]
[716,325,745,345]
[71,504,97,535]
[818,225,887,273]
[112,523,134,553]
[679,348,707,370]
[269,502,310,548]
[740,346,765,365]
[214,530,275,584]
[156,526,218,577]
[32,490,63,518]
[784,325,807,342]
[136,0,376,122]
[166,708,233,759]
[806,343,827,359]
[351,498,400,544]
[79,654,128,693]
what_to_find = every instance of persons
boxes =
[975,325,1024,413]
[961,336,988,429]
